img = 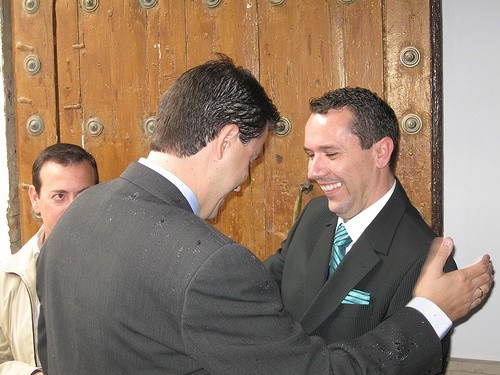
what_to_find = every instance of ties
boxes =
[329,223,353,279]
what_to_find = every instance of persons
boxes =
[263,86,458,375]
[0,143,100,375]
[36,52,494,375]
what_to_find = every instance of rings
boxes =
[478,287,483,298]
